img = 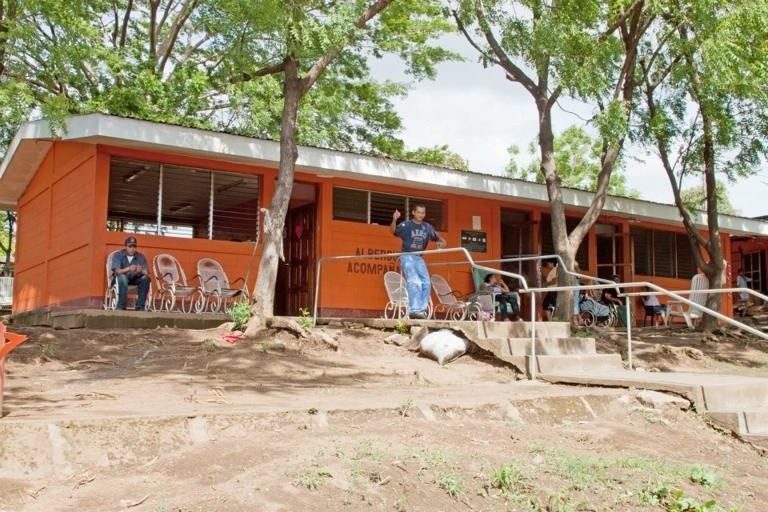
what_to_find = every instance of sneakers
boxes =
[409,312,425,319]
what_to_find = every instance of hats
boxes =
[126,237,138,247]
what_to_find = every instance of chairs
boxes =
[577,274,710,332]
[102,248,250,315]
[383,264,521,321]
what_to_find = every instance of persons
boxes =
[736,268,759,301]
[390,202,447,320]
[112,237,151,311]
[542,257,631,328]
[643,281,671,328]
[480,273,525,322]
